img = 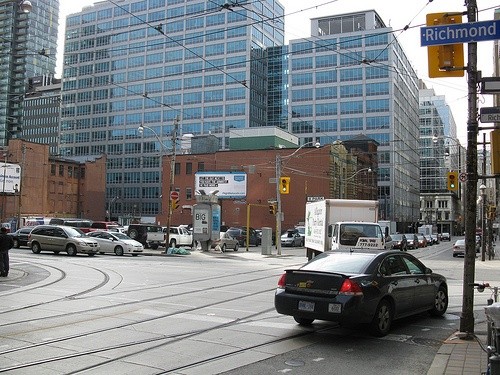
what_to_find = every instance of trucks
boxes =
[305,198,393,262]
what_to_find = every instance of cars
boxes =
[7,219,198,257]
[452,228,482,257]
[274,249,449,338]
[377,220,451,251]
[281,226,305,246]
[212,226,274,252]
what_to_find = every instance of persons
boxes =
[0,227,14,277]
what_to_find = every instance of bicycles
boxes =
[468,282,500,375]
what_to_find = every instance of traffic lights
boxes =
[269,205,275,216]
[280,177,290,194]
[447,172,458,190]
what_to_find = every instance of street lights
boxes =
[138,124,176,255]
[275,139,320,256]
[433,135,487,261]
[341,167,371,198]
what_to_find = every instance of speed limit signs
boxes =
[170,191,179,200]
